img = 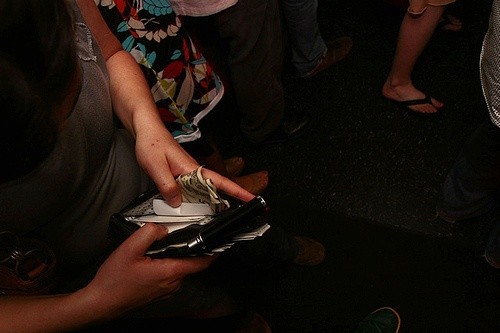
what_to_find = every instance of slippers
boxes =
[437,14,464,32]
[380,89,449,120]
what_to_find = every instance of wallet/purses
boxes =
[110,177,266,250]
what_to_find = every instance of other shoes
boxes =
[484,222,500,268]
[230,170,270,195]
[354,307,401,333]
[231,310,272,333]
[301,35,355,81]
[219,155,246,177]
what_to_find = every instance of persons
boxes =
[0,0,463,333]
[434,0,500,272]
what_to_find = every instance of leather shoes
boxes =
[252,112,312,148]
